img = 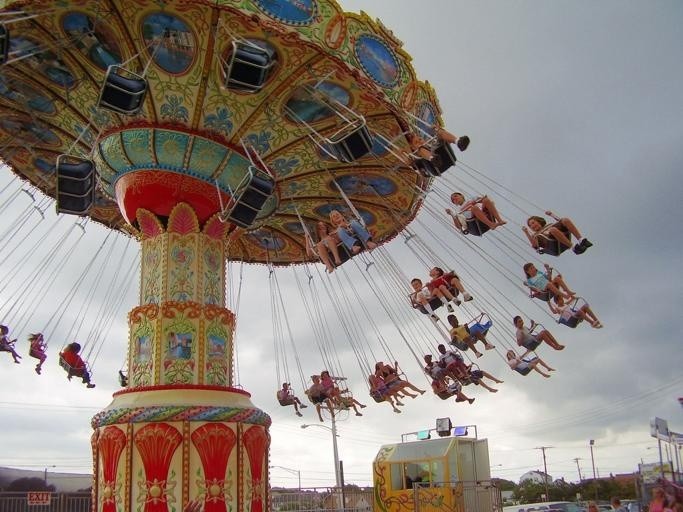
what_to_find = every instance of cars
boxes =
[502,498,643,512]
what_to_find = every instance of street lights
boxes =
[589,438,601,503]
[271,464,301,492]
[300,421,343,510]
[489,463,502,468]
[43,465,56,481]
[646,446,665,450]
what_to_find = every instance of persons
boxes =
[0,324,22,366]
[60,342,95,388]
[546,294,602,330]
[609,496,628,512]
[521,209,594,256]
[28,332,48,374]
[280,382,306,418]
[512,315,566,350]
[505,349,557,380]
[648,484,683,511]
[519,260,576,301]
[444,190,506,235]
[405,126,471,177]
[588,502,600,512]
[405,469,425,489]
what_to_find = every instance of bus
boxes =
[269,489,312,510]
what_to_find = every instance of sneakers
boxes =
[463,294,473,302]
[395,401,404,406]
[430,314,439,323]
[474,351,482,359]
[590,320,603,329]
[393,408,401,414]
[446,304,454,312]
[456,134,469,152]
[571,243,585,255]
[454,397,475,405]
[360,404,367,409]
[580,238,593,248]
[453,300,460,307]
[355,412,363,417]
[295,404,308,418]
[428,153,442,168]
[484,344,495,350]
